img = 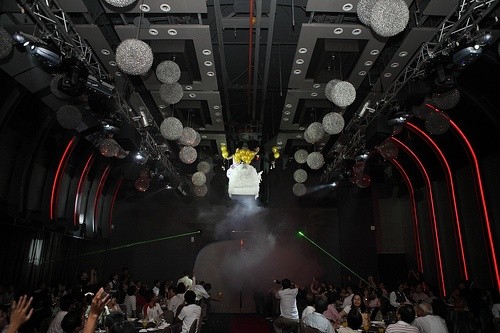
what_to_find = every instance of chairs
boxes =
[162,310,174,326]
[119,304,127,321]
[188,319,198,333]
[174,304,188,324]
[299,327,320,333]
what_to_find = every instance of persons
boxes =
[0,262,453,333]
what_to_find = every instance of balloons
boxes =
[221,140,282,166]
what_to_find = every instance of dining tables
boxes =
[443,303,480,333]
[340,320,395,333]
[96,318,172,333]
